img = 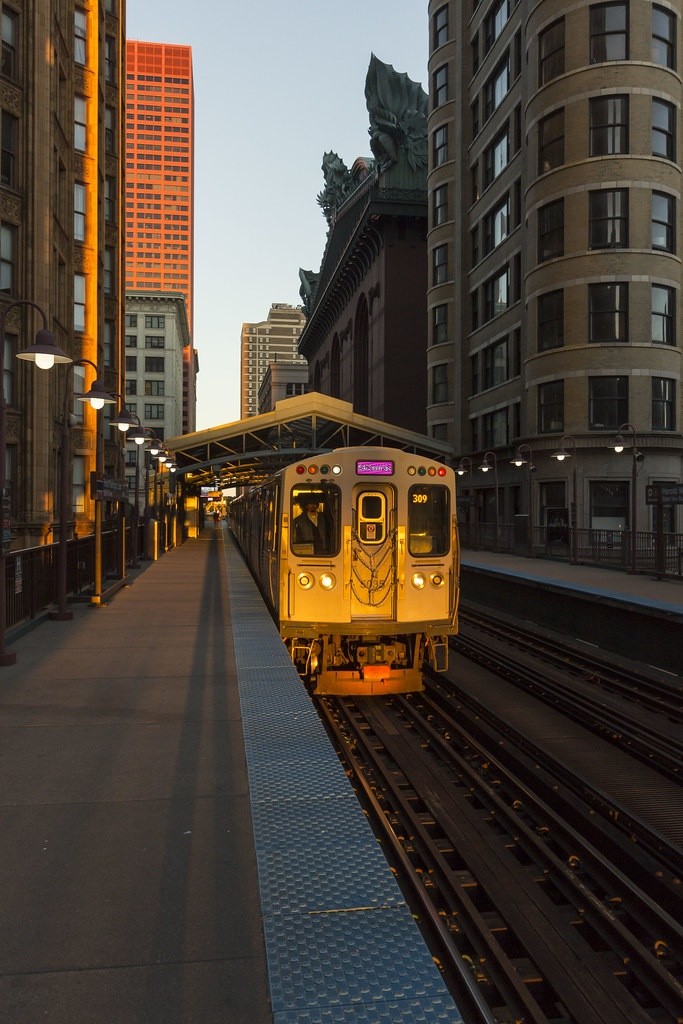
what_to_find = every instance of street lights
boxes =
[476,452,504,554]
[41,358,118,623]
[91,392,140,609]
[606,423,644,576]
[0,299,75,674]
[550,436,583,566]
[509,444,536,559]
[455,457,472,550]
[125,426,181,579]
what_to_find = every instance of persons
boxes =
[294,495,327,555]
[213,510,219,528]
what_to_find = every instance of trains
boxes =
[226,446,462,698]
